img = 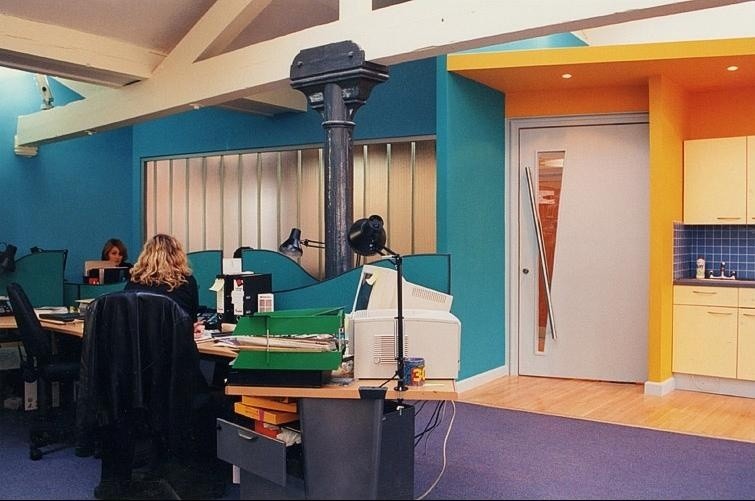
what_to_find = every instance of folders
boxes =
[212,272,273,332]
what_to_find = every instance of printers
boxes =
[350,309,461,381]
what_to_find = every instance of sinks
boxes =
[701,279,740,281]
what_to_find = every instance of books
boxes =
[234,396,299,425]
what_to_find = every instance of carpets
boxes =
[2,398,754,500]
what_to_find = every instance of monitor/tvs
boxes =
[351,263,454,313]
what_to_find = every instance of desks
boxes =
[1,307,458,501]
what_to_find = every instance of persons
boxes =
[233,246,254,258]
[123,234,199,326]
[101,239,132,268]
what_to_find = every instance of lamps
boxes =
[346,215,409,394]
[279,228,327,260]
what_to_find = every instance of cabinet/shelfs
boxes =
[216,401,415,500]
[682,134,755,226]
[672,285,755,382]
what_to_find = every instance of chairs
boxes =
[79,291,214,501]
[3,283,86,464]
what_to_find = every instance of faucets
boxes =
[721,260,726,277]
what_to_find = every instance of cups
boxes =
[404,358,424,387]
